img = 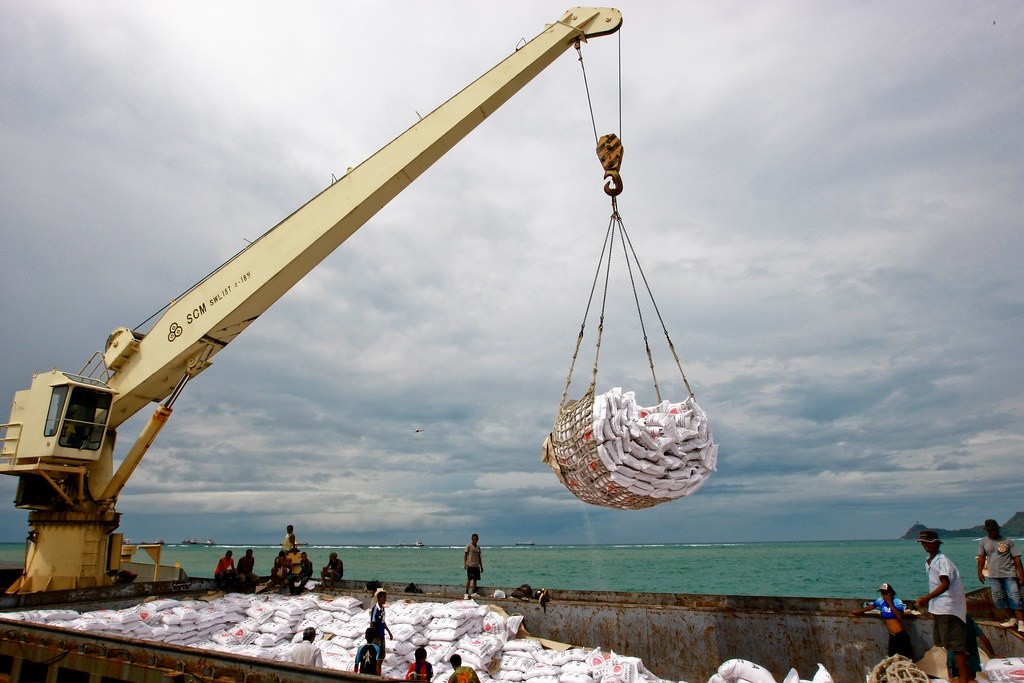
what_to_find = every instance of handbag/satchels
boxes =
[493,590,506,598]
[405,583,422,593]
[535,590,549,601]
[367,580,381,590]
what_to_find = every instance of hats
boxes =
[303,627,319,636]
[984,520,1001,529]
[279,550,285,557]
[917,530,943,544]
[877,583,892,592]
[300,552,307,557]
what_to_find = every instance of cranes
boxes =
[0,6,628,593]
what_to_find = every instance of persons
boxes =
[448,654,481,683]
[851,582,915,663]
[946,613,1007,679]
[318,552,343,592]
[405,648,434,682]
[370,591,393,664]
[288,627,323,668]
[914,530,970,683]
[214,550,240,594]
[268,525,313,596]
[463,534,484,600]
[236,549,260,594]
[354,628,384,676]
[978,519,1024,632]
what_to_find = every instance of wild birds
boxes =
[413,429,423,432]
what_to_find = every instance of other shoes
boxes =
[1018,624,1024,632]
[1000,621,1017,627]
[464,594,468,599]
[471,593,481,597]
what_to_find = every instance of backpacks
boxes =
[512,584,532,598]
